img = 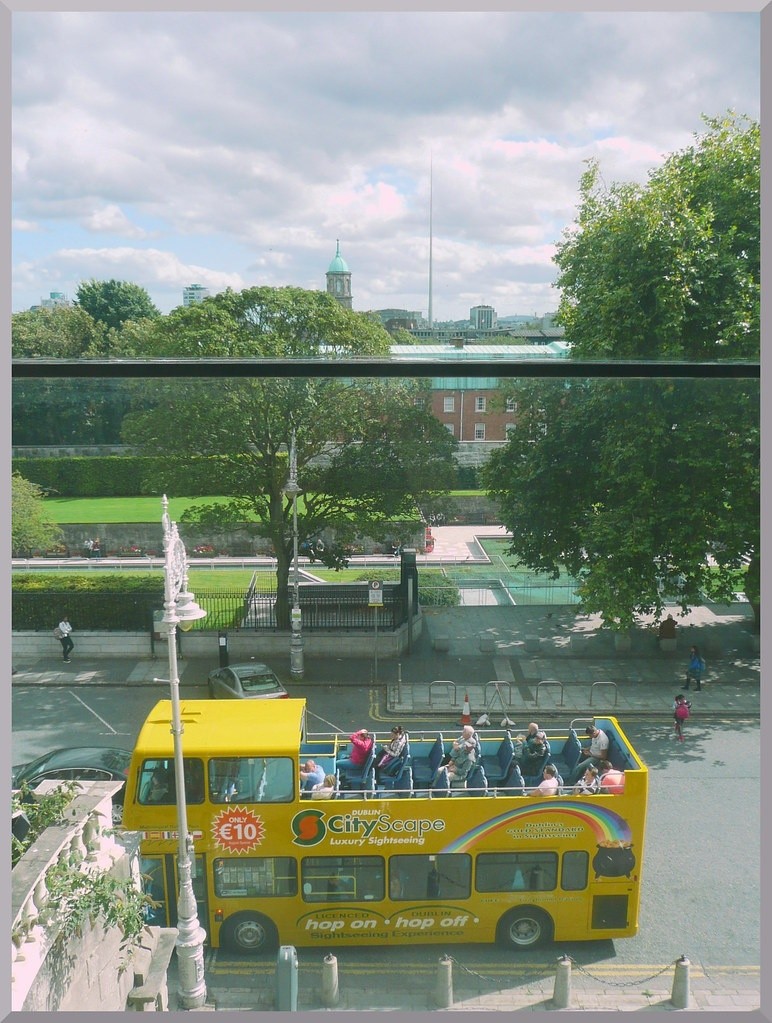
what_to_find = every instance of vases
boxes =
[119,552,141,556]
[194,552,215,558]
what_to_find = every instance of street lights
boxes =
[284,428,305,680]
[154,493,207,1008]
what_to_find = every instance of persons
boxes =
[310,539,324,562]
[657,615,678,639]
[682,646,702,690]
[83,538,100,557]
[215,758,241,802]
[526,767,558,796]
[148,772,169,801]
[428,511,445,527]
[370,727,406,773]
[510,723,547,777]
[392,538,401,557]
[59,617,74,663]
[599,760,613,793]
[674,696,691,744]
[428,726,476,789]
[336,728,372,769]
[568,725,609,786]
[572,767,598,795]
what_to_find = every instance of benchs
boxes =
[82,543,107,557]
[454,512,496,526]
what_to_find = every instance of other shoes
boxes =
[681,686,688,690]
[674,723,678,732]
[693,688,701,691]
[678,735,683,741]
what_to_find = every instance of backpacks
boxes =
[385,756,403,775]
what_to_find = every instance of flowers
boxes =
[191,544,217,558]
[119,544,141,552]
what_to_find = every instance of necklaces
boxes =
[300,760,336,800]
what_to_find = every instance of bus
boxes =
[122,697,650,956]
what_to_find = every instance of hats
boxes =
[465,738,476,748]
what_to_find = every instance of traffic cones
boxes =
[456,695,476,727]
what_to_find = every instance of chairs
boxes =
[336,727,632,800]
[254,759,266,801]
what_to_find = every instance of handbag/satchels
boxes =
[378,754,393,768]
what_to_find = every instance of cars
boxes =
[12,747,133,825]
[206,662,289,700]
